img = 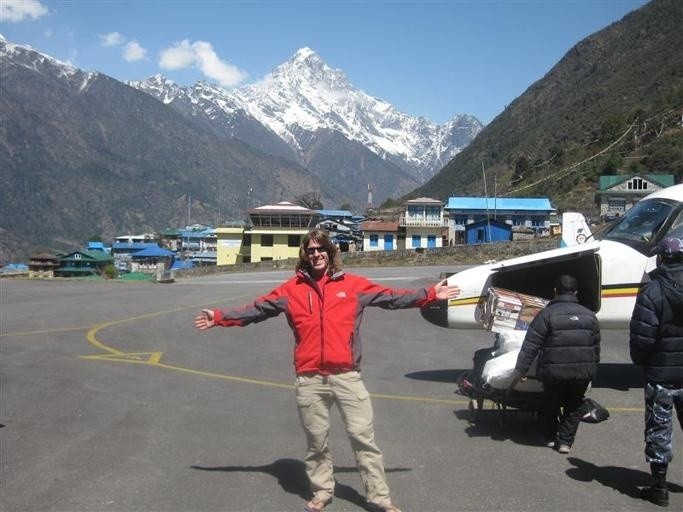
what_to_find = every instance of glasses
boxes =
[305,247,327,254]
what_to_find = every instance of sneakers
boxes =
[557,444,571,453]
[542,438,555,447]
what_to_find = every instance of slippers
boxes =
[305,498,332,512]
[367,503,401,512]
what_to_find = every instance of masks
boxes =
[656,256,663,268]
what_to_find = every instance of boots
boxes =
[642,468,668,507]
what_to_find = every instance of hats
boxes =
[651,237,683,254]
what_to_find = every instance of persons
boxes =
[629,237,683,507]
[195,229,462,512]
[514,275,602,454]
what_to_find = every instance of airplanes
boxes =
[419,183,683,365]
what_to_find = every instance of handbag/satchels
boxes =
[581,399,609,423]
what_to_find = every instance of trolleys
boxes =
[462,347,561,433]
